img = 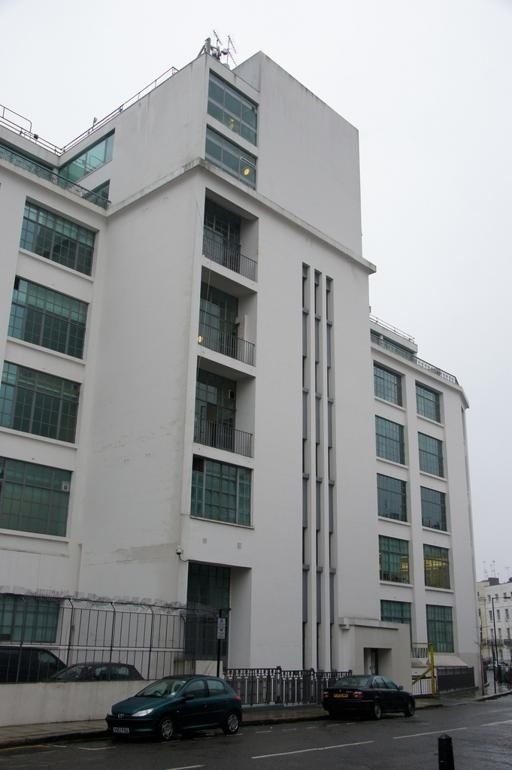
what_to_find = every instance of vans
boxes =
[0,646,68,683]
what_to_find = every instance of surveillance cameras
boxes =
[176,544,184,557]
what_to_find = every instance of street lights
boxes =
[491,596,511,692]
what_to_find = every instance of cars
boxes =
[41,662,146,681]
[322,675,415,721]
[483,654,512,684]
[104,673,243,742]
